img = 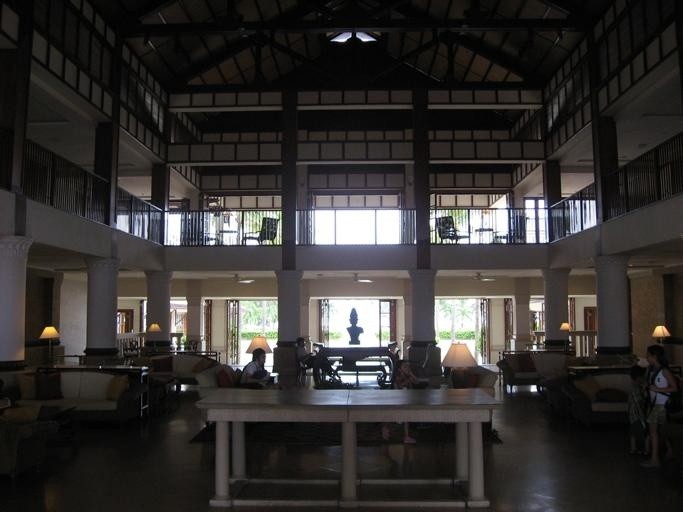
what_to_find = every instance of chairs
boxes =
[495,216,528,245]
[436,216,458,244]
[182,218,215,245]
[496,348,683,440]
[193,362,495,446]
[241,217,279,246]
[2,354,223,476]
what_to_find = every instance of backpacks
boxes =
[647,364,683,413]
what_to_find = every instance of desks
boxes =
[474,228,493,244]
[196,386,504,511]
[218,231,239,246]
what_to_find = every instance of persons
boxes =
[239,348,272,389]
[296,337,341,380]
[627,365,648,455]
[380,360,420,444]
[387,342,400,362]
[637,344,679,470]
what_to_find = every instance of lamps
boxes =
[38,326,62,364]
[559,322,571,353]
[652,325,671,354]
[147,324,163,353]
